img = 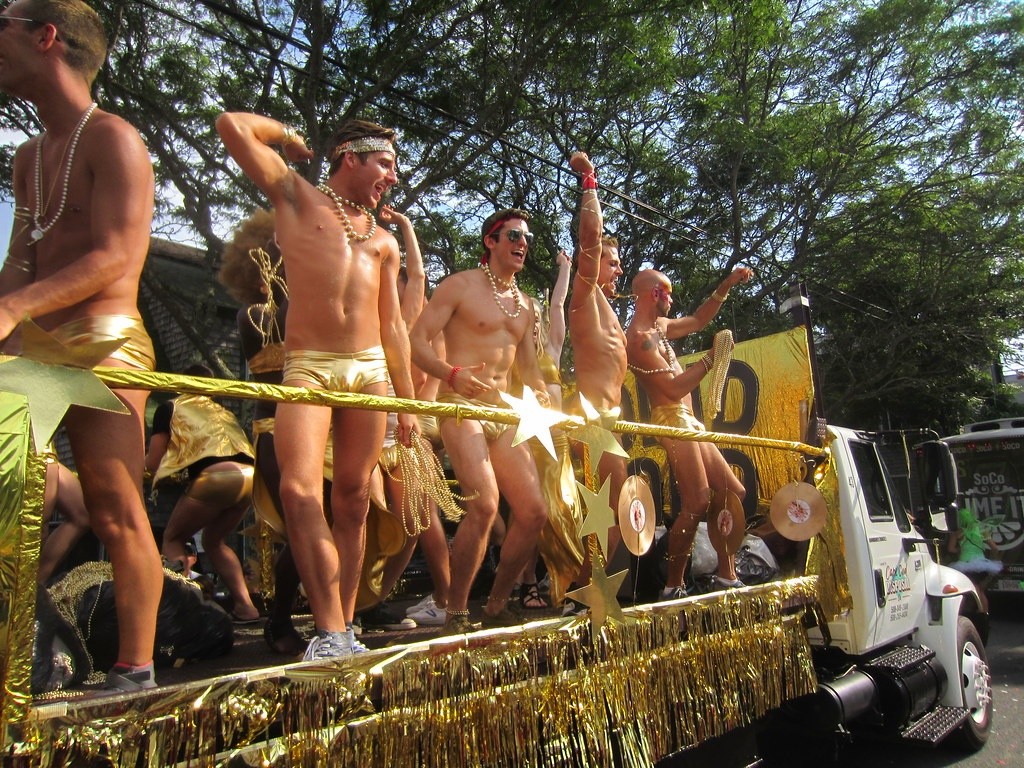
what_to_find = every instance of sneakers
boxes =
[301,621,371,662]
[359,605,417,632]
[71,660,159,704]
[405,592,448,626]
[709,574,747,591]
[656,584,688,602]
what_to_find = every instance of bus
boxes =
[935,418,1024,592]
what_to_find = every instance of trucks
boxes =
[0,283,996,768]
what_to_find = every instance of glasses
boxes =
[489,228,534,245]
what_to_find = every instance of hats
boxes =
[185,541,197,555]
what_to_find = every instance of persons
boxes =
[946,509,1002,609]
[42,112,628,685]
[0,0,164,693]
[627,266,755,600]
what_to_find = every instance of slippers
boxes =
[228,612,261,625]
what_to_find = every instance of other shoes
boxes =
[479,606,524,626]
[263,618,309,658]
[444,609,477,634]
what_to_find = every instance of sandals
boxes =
[519,582,547,610]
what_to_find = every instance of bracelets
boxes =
[143,467,157,479]
[283,125,296,146]
[448,367,461,387]
[701,354,713,372]
[712,290,728,302]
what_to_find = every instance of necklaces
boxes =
[626,324,675,373]
[31,102,96,231]
[381,422,479,537]
[319,183,376,241]
[708,330,733,418]
[482,263,521,318]
